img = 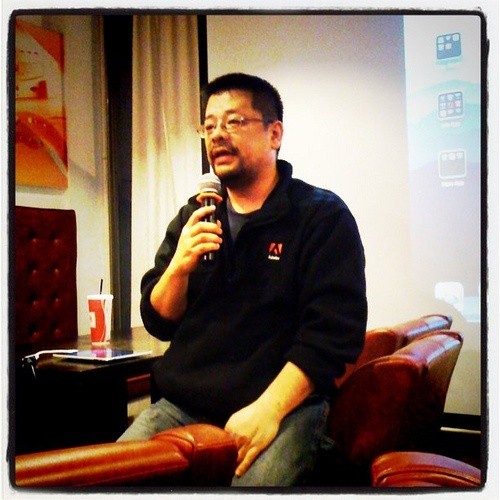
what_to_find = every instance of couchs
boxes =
[13,312,481,489]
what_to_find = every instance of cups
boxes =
[89,295,113,346]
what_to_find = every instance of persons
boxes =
[116,71,369,486]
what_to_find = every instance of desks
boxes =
[12,327,172,454]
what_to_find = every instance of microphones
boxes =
[198,173,222,262]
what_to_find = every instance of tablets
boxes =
[52,348,153,361]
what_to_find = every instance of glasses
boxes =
[194,117,274,138]
